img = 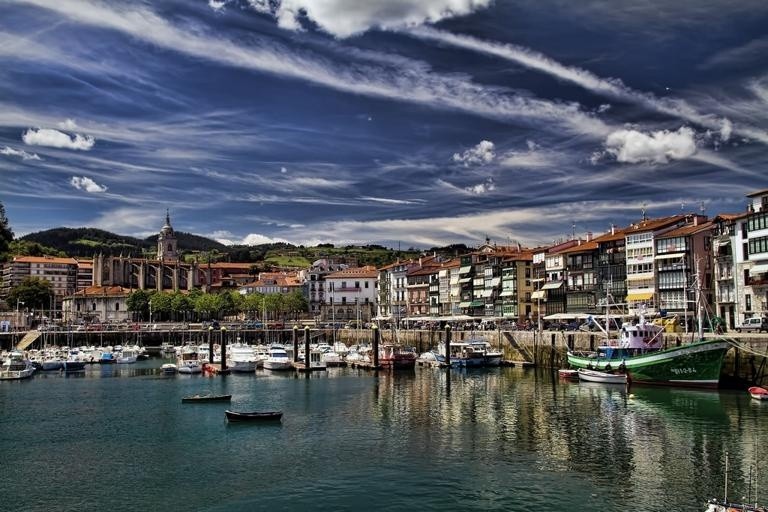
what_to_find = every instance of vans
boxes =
[736,318,767,335]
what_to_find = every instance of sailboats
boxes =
[0,298,149,379]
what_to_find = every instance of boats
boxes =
[225,409,283,422]
[558,256,738,390]
[747,384,768,400]
[182,394,236,405]
